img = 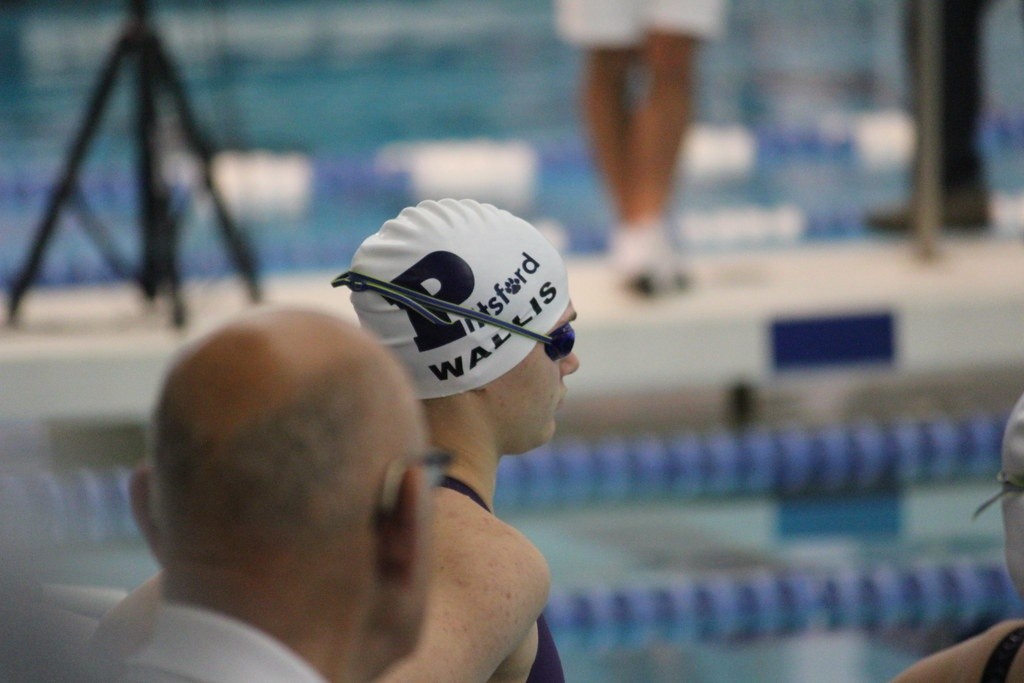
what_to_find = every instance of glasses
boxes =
[377,443,452,499]
[330,267,578,364]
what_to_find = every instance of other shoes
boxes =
[608,214,683,298]
[868,189,985,234]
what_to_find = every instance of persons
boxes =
[331,198,580,683]
[552,0,731,305]
[888,390,1024,683]
[72,308,431,683]
[863,0,998,237]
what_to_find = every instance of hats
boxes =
[346,198,569,400]
